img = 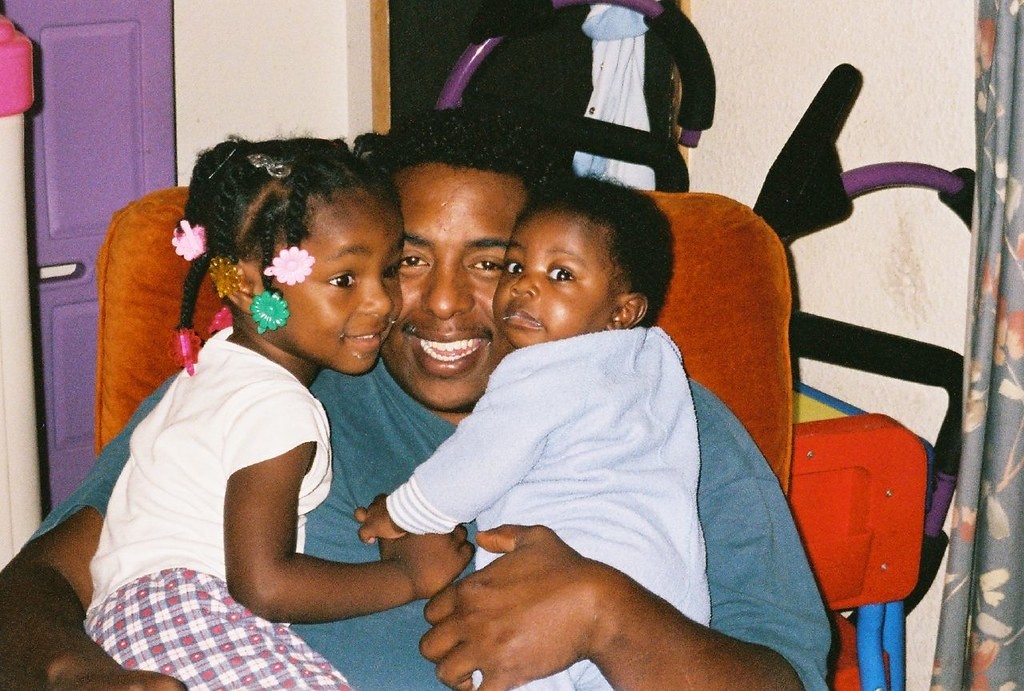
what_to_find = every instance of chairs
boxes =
[787,411,923,691]
[95,177,792,520]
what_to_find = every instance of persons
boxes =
[84,134,476,691]
[350,169,711,691]
[0,107,835,691]
